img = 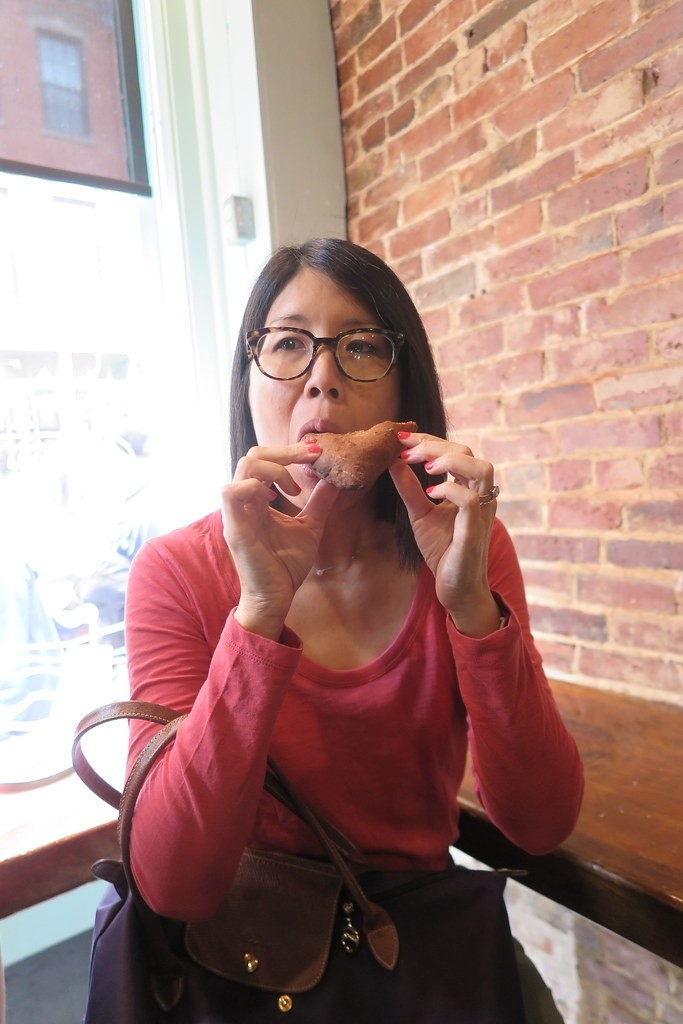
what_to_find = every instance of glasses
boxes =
[244,327,409,383]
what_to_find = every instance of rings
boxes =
[479,486,500,506]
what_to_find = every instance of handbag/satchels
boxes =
[72,703,529,1024]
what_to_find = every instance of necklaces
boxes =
[314,525,386,576]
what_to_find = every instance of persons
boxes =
[128,239,584,919]
